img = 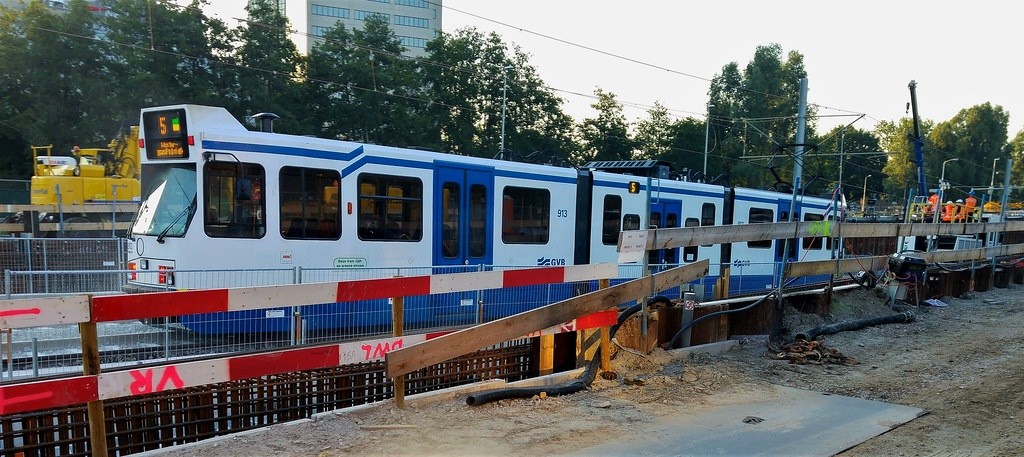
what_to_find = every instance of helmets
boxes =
[969,191,975,195]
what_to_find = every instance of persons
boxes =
[964,190,977,223]
[940,199,966,223]
[922,189,942,222]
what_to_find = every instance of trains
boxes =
[122,103,850,345]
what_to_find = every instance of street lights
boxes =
[940,158,959,204]
[861,174,872,212]
[989,158,1000,202]
[499,66,514,160]
[703,101,716,176]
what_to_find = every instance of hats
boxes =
[956,199,963,202]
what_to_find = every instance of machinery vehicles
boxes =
[0,122,140,270]
[906,78,979,224]
